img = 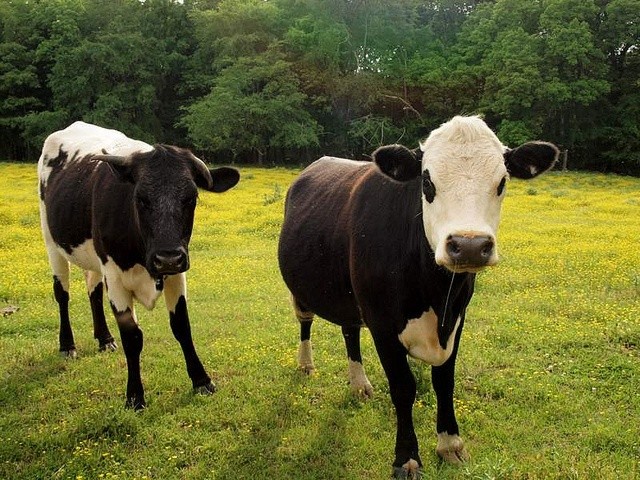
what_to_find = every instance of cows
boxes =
[37,121,241,412]
[278,112,561,480]
